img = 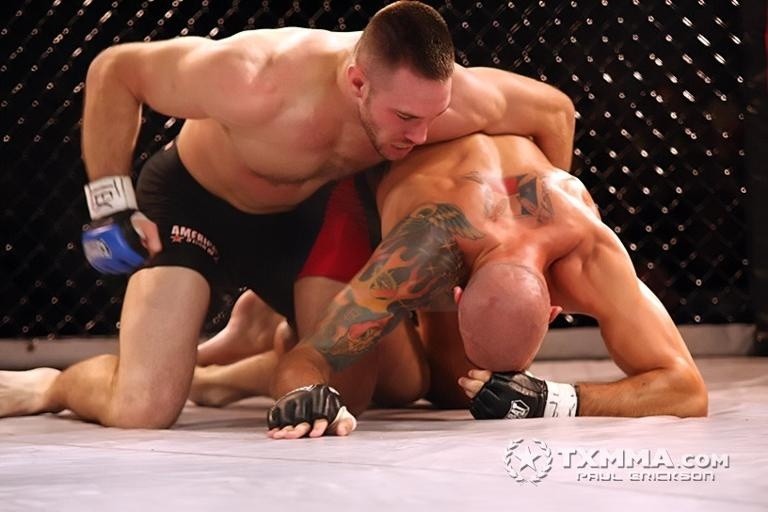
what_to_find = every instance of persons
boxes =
[190,133,708,441]
[0,0,576,429]
